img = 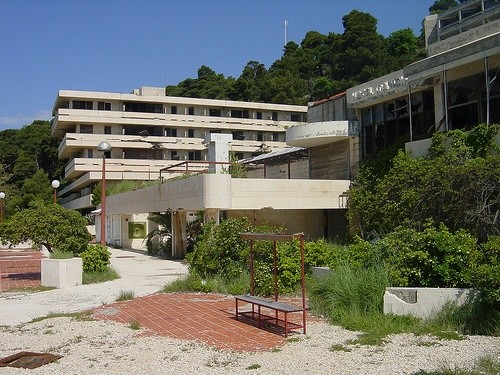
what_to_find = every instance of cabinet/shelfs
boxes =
[235,293,305,337]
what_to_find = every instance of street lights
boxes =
[0,192,7,224]
[97,140,110,246]
[50,179,60,205]
